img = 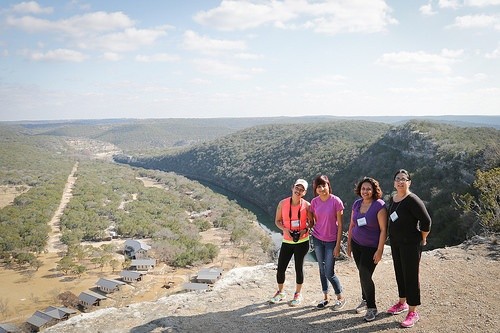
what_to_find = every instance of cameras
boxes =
[290,229,300,242]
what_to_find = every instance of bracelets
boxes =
[303,227,308,234]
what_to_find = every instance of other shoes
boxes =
[332,297,346,311]
[316,299,330,310]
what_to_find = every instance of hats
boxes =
[294,179,308,190]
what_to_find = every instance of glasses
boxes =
[395,177,410,182]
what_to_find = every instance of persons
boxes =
[348,176,387,321]
[387,169,431,328]
[270,179,311,306]
[308,175,346,310]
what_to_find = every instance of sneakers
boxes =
[387,302,409,314]
[401,309,420,328]
[268,291,286,304]
[290,291,302,306]
[363,308,379,321]
[355,299,368,314]
